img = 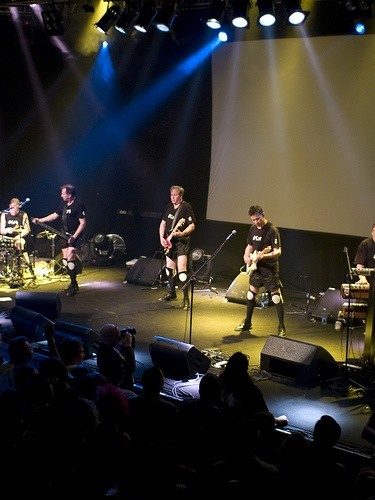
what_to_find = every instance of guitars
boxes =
[246,246,272,276]
[30,216,90,261]
[160,217,185,256]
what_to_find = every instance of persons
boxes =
[158,185,196,311]
[1,325,375,500]
[32,184,87,297]
[0,197,35,283]
[237,205,287,339]
[353,224,375,288]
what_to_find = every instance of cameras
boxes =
[120,327,137,338]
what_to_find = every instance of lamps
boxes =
[156,0,184,32]
[115,0,138,34]
[189,248,212,276]
[90,233,113,260]
[206,0,228,29]
[41,2,64,36]
[255,0,276,26]
[281,0,311,26]
[94,0,120,34]
[228,0,251,28]
[134,0,159,33]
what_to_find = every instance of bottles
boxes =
[321,307,328,325]
[260,293,269,308]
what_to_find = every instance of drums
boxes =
[0,238,15,251]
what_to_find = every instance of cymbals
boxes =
[37,231,55,239]
[13,228,29,233]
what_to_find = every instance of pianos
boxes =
[341,302,368,320]
[340,283,370,301]
[347,267,375,277]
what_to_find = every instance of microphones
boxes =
[226,229,237,241]
[20,197,30,207]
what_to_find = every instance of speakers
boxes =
[312,287,364,326]
[260,333,341,389]
[10,305,56,343]
[123,257,169,287]
[52,321,103,362]
[224,271,273,306]
[149,335,211,380]
[14,290,62,319]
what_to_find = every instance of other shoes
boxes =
[164,293,176,300]
[64,281,78,296]
[278,325,286,336]
[235,323,253,331]
[182,302,189,310]
